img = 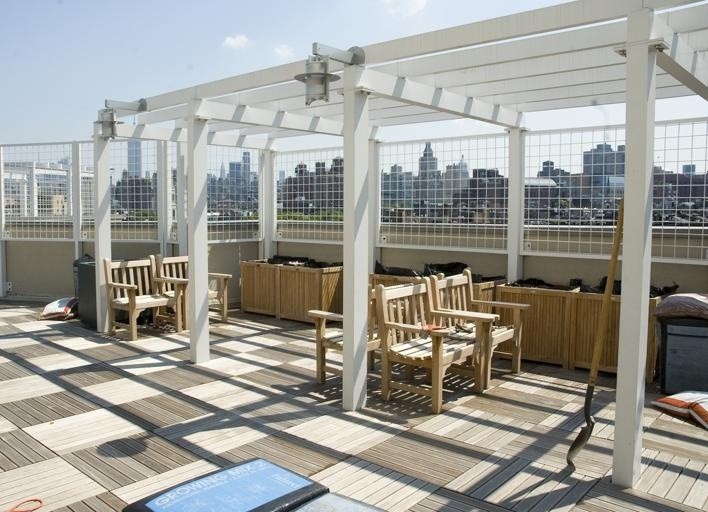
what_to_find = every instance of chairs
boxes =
[307,268,530,413]
[103,254,187,342]
[156,253,233,330]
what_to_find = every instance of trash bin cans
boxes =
[77,259,124,329]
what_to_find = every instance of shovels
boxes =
[567,197,624,465]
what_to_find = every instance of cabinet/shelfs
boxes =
[495,282,571,369]
[569,286,665,384]
[240,259,278,319]
[278,266,343,324]
